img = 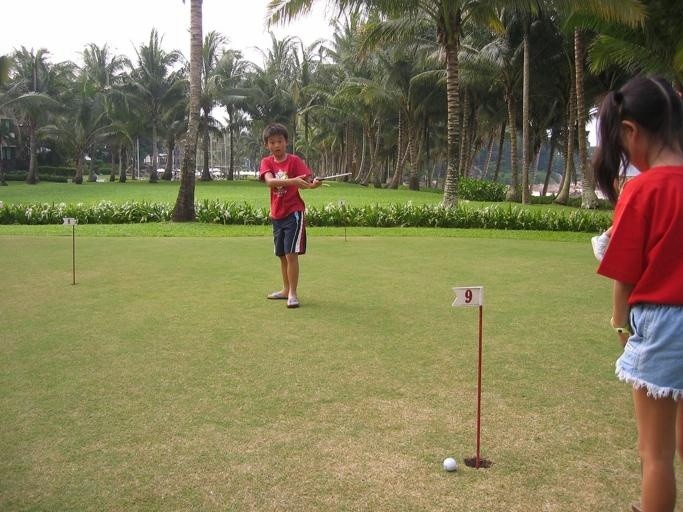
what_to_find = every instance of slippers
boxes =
[267,292,299,308]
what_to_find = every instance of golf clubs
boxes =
[304,160,355,182]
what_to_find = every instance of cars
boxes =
[212,169,224,178]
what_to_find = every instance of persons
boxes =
[258,123,323,308]
[588,73,683,512]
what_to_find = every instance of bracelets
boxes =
[608,317,631,335]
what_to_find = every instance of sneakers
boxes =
[591,233,610,261]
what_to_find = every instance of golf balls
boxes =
[441,455,458,474]
